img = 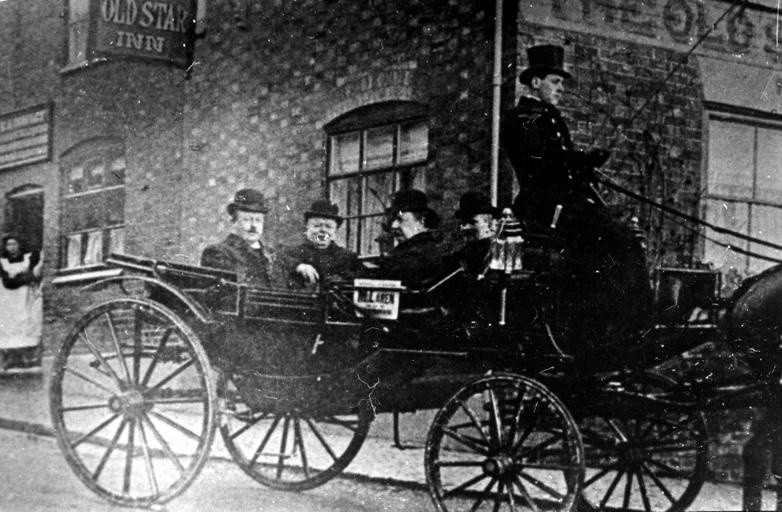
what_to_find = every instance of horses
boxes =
[722,264,782,512]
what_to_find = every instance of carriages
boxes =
[48,202,782,512]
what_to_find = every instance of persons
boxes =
[202,188,321,290]
[0,235,44,369]
[434,192,499,274]
[276,198,368,290]
[373,189,441,284]
[502,43,659,338]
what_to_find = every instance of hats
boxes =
[454,190,501,218]
[303,199,343,222]
[520,44,571,80]
[227,188,268,215]
[386,188,440,230]
[2,233,23,244]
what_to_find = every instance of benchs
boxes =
[131,187,466,325]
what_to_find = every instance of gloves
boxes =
[296,263,320,285]
